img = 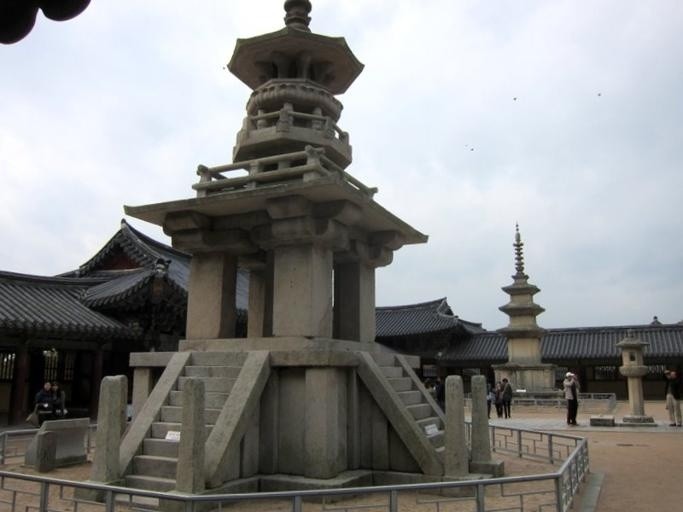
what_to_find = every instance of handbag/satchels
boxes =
[26,412,40,427]
[499,392,503,400]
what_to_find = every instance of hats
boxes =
[566,372,574,377]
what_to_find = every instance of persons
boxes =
[486,379,491,419]
[435,375,445,412]
[663,369,681,427]
[562,371,580,426]
[500,378,512,418]
[51,381,72,419]
[34,381,55,426]
[493,382,503,416]
